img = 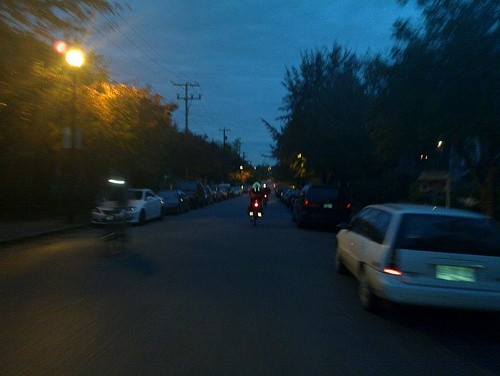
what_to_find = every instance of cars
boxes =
[281,187,289,200]
[285,189,298,202]
[243,183,251,192]
[230,187,243,197]
[210,185,228,202]
[275,187,285,198]
[201,184,215,206]
[290,189,304,209]
[91,187,166,225]
[157,189,190,214]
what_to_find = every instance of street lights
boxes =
[66,40,88,222]
[296,151,304,181]
[239,164,245,187]
[108,173,126,187]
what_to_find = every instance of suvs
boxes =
[173,181,205,209]
[293,182,353,231]
[334,204,499,312]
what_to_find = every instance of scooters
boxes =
[247,195,265,226]
[262,192,270,208]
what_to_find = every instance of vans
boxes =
[219,183,231,200]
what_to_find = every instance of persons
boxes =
[247,182,265,224]
